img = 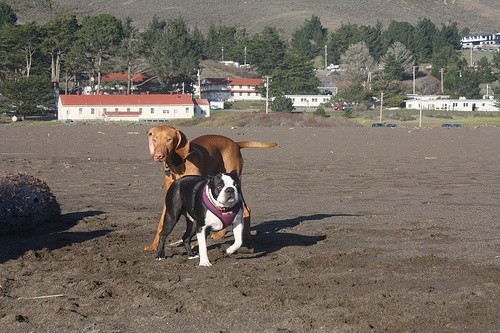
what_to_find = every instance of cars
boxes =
[372,122,397,129]
[441,123,465,128]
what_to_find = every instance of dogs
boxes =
[143,125,278,268]
